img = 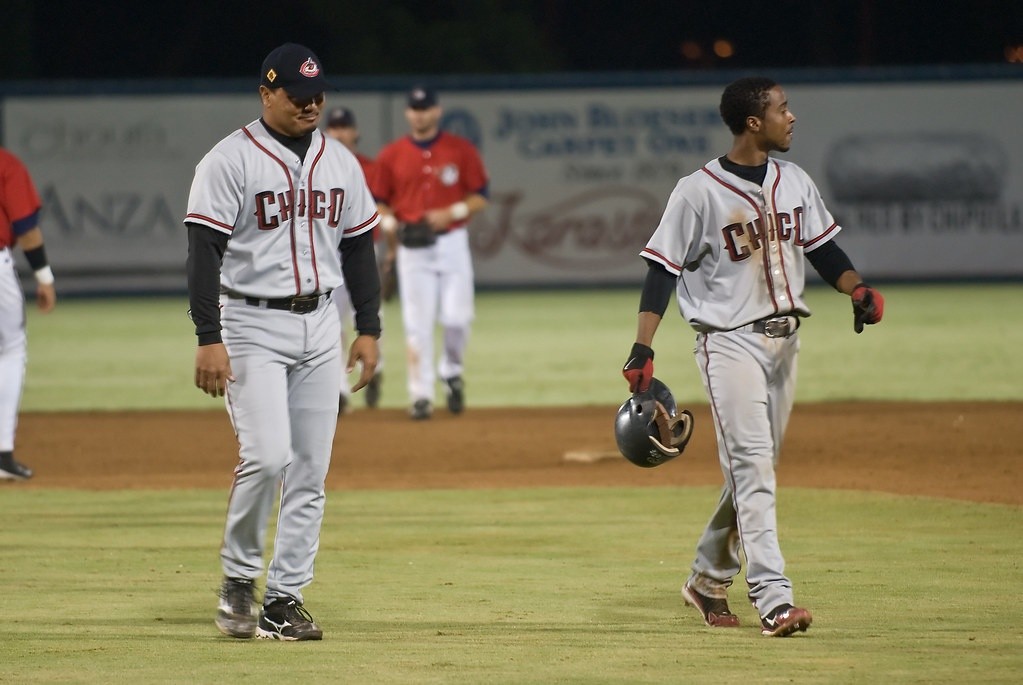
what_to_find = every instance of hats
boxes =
[409,86,435,109]
[261,43,324,100]
[326,107,356,128]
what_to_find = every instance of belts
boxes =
[246,290,330,314]
[752,316,801,337]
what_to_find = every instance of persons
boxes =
[623,78,883,637]
[0,148,56,483]
[373,86,490,420]
[183,44,382,642]
[323,105,397,418]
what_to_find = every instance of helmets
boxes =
[615,377,694,468]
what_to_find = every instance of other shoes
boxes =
[448,378,464,414]
[414,399,434,418]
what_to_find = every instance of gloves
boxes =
[622,342,654,392]
[850,285,884,334]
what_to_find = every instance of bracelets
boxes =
[450,203,469,220]
[24,245,47,270]
[380,216,395,231]
[36,266,54,284]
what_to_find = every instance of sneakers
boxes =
[0,463,32,479]
[214,575,262,639]
[682,582,739,627]
[254,597,323,641]
[761,603,812,636]
